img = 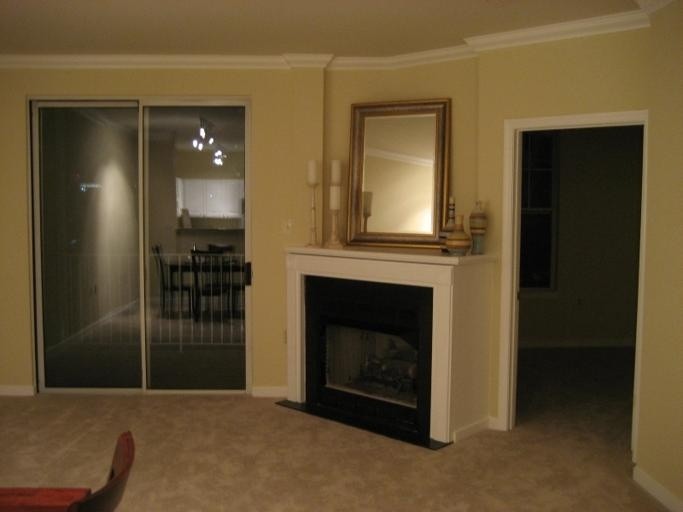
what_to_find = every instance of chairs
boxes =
[155,245,237,320]
[77,429,134,512]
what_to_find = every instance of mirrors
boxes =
[344,98,454,250]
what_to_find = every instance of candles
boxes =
[328,160,342,211]
[307,162,316,186]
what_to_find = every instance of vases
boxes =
[440,197,486,256]
